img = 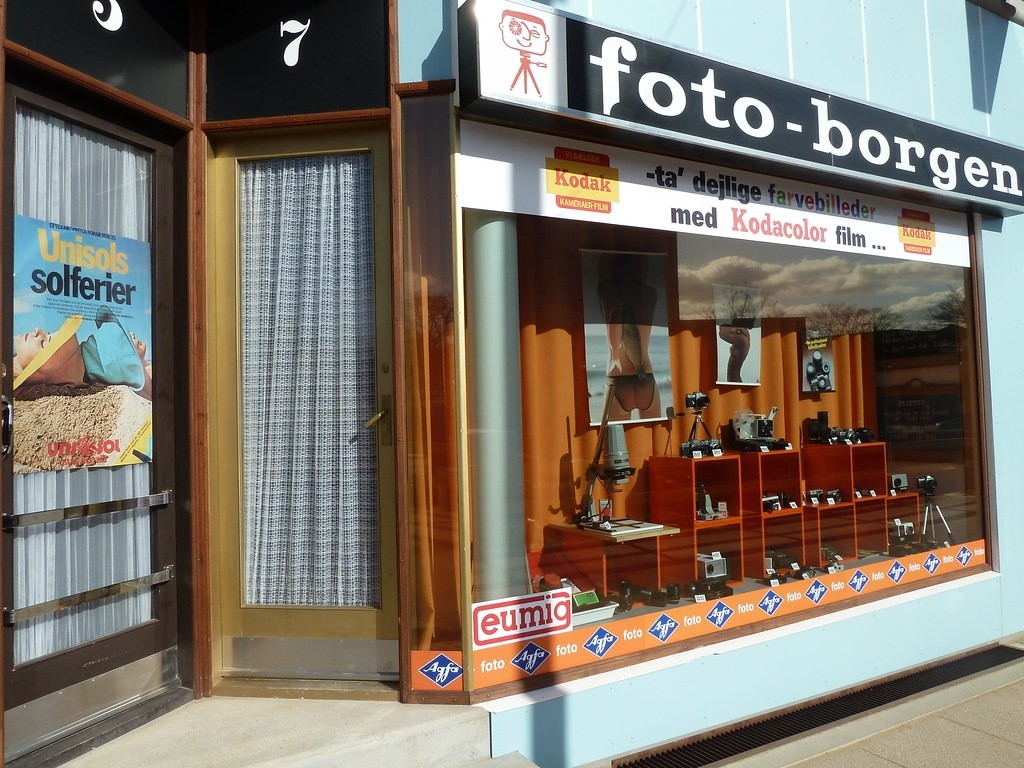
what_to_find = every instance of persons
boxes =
[597,253,662,421]
[81,305,152,396]
[715,286,754,383]
[13,315,85,391]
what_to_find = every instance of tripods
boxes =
[676,409,713,442]
[921,495,956,549]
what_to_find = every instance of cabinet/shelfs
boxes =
[649,454,742,594]
[805,442,919,566]
[548,520,681,599]
[724,447,806,578]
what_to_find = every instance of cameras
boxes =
[827,489,841,503]
[685,392,710,408]
[809,411,873,446]
[807,488,824,502]
[762,491,792,513]
[917,476,937,494]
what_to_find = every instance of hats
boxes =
[13,313,84,391]
[80,305,146,393]
